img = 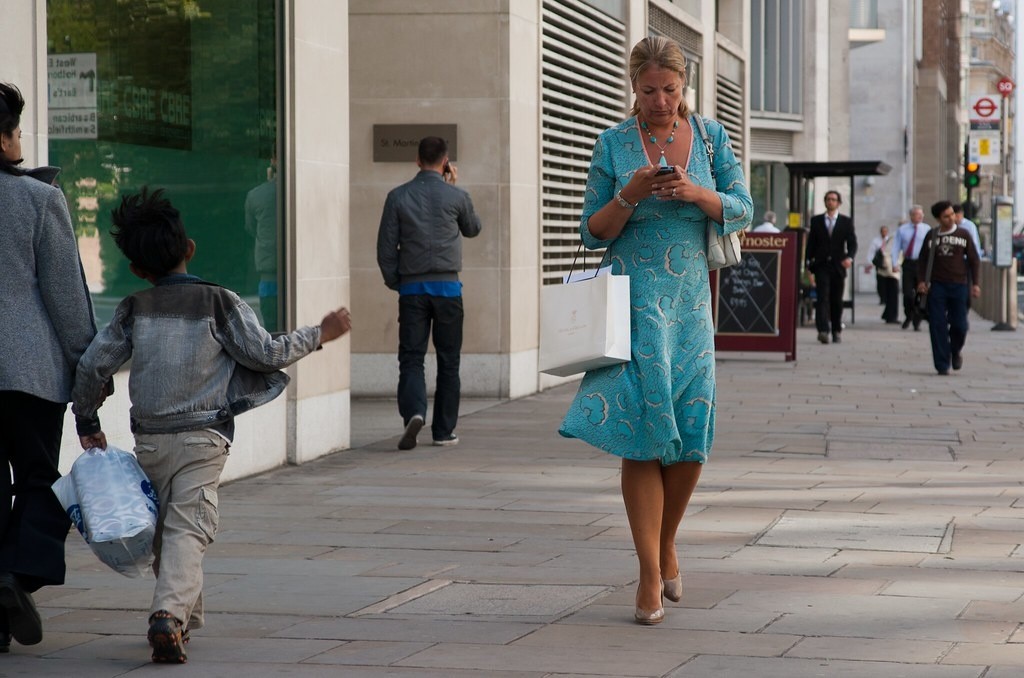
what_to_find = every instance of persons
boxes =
[243,138,280,333]
[754,211,781,233]
[377,136,482,450]
[805,191,858,342]
[72,185,352,663]
[864,205,981,331]
[915,199,981,374]
[559,37,753,624]
[0,84,115,654]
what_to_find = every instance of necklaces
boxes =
[639,114,679,167]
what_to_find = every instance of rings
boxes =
[672,189,676,196]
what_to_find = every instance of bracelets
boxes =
[616,190,638,208]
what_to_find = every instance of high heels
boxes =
[662,572,682,601]
[635,577,665,624]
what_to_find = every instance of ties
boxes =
[905,224,917,256]
[828,217,834,238]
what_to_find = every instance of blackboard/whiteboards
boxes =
[708,231,797,352]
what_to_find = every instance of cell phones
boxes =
[655,166,674,176]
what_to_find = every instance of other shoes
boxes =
[833,331,841,342]
[147,610,190,663]
[399,414,424,450]
[433,433,458,445]
[902,317,911,329]
[914,323,920,331]
[818,330,829,344]
[952,351,962,369]
[0,572,42,646]
[938,369,949,375]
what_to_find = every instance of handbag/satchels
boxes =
[873,248,886,270]
[51,443,160,578]
[539,235,631,377]
[693,112,742,271]
[917,293,927,312]
